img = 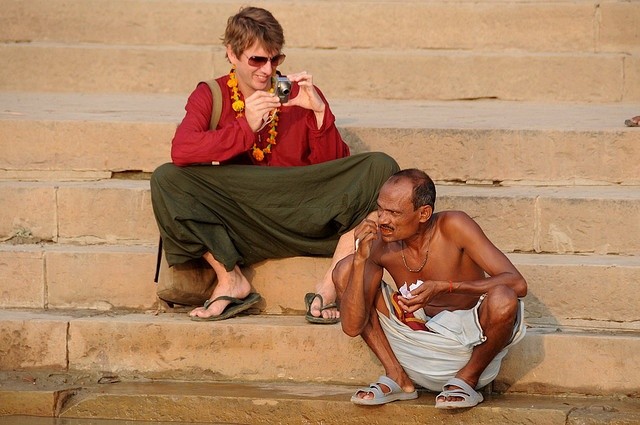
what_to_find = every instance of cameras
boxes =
[276,76,292,104]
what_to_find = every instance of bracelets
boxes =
[446,279,453,295]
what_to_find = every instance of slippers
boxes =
[305,292,341,324]
[188,292,262,321]
[350,375,419,405]
[435,377,483,409]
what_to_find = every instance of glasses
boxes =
[242,52,286,66]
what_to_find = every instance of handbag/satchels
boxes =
[156,235,217,307]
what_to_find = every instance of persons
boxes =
[149,6,402,325]
[330,167,530,412]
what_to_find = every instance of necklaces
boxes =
[227,64,282,162]
[400,213,434,273]
